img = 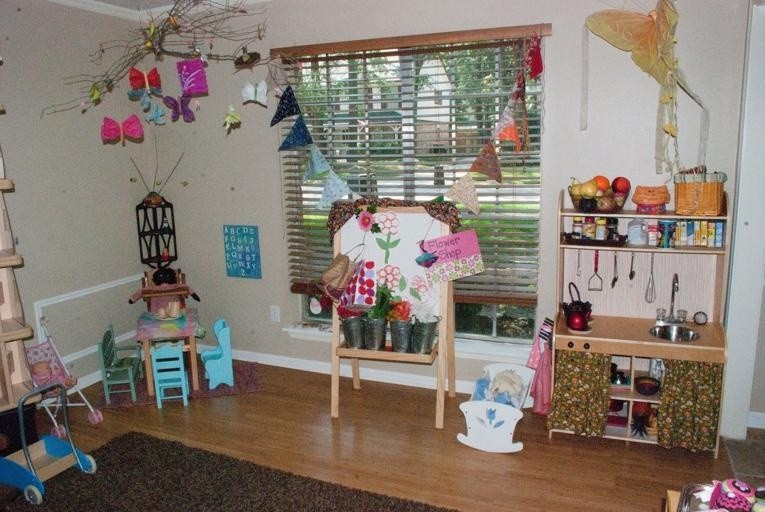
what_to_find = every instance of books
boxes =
[675,220,723,248]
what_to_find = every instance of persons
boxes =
[31,360,78,397]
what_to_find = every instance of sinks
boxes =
[649,324,701,341]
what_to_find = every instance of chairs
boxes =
[148,342,188,410]
[201,318,235,390]
[99,326,144,404]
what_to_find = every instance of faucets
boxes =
[667,273,679,320]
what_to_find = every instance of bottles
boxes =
[572,216,618,242]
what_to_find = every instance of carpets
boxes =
[7,430,461,511]
[99,360,256,407]
[726,435,763,511]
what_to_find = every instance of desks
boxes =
[136,312,202,394]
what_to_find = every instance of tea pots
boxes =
[563,282,591,331]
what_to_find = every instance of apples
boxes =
[612,177,630,194]
[597,196,616,212]
[571,184,582,195]
[579,181,597,200]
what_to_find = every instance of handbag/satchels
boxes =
[316,244,365,303]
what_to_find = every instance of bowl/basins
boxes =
[610,371,627,384]
[634,376,661,396]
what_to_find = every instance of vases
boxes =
[340,316,438,353]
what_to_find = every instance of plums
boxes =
[579,198,596,212]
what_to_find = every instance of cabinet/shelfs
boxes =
[558,195,731,254]
[552,351,722,453]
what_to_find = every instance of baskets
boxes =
[674,180,724,216]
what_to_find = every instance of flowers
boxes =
[319,279,412,321]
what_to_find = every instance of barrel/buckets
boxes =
[627,217,649,248]
[338,315,442,356]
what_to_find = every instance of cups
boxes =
[157,301,181,319]
